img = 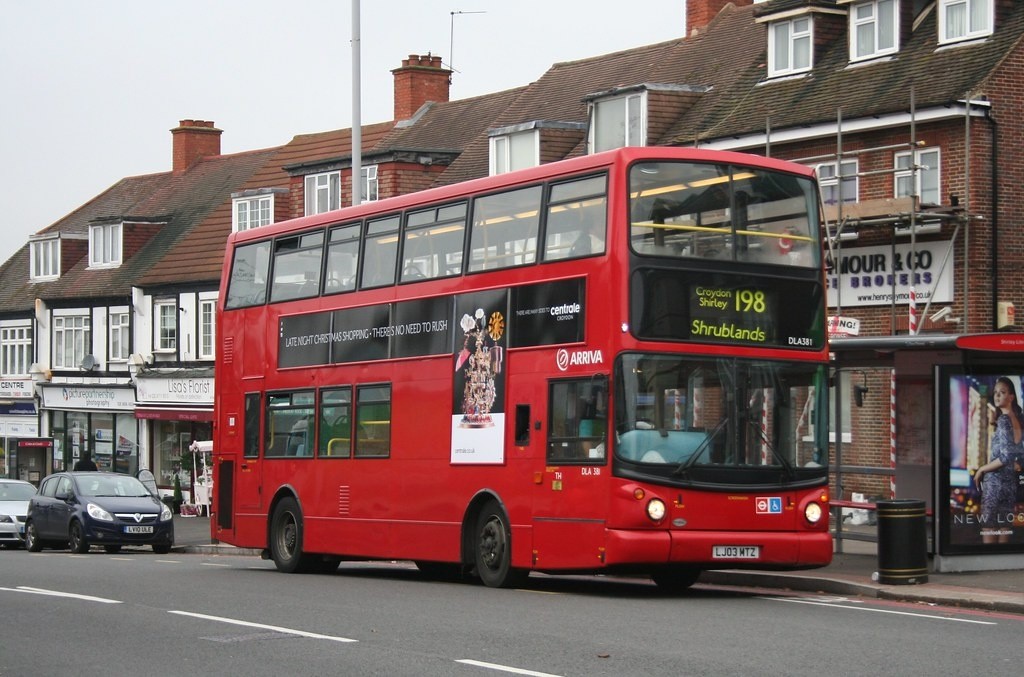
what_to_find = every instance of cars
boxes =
[25,471,175,554]
[0,478,39,551]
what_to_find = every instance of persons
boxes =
[973,378,1024,544]
[73,450,99,472]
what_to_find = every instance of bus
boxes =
[213,145,833,595]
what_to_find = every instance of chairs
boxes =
[286,414,369,456]
[96,482,116,496]
[569,236,592,256]
[580,386,619,458]
[252,266,456,305]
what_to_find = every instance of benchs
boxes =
[828,499,932,517]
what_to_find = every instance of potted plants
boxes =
[173,475,185,514]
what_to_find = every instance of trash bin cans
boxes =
[875,499,929,586]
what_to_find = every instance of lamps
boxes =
[81,354,100,371]
